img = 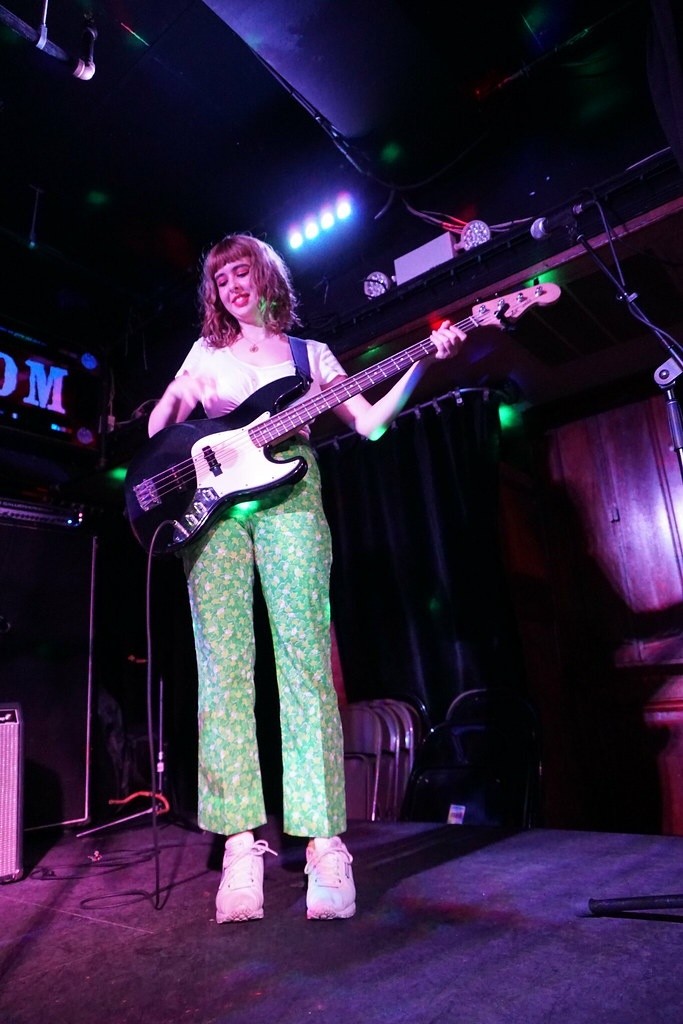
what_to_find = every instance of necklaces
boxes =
[243,332,274,352]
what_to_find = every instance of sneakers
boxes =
[305,837,359,919]
[217,835,267,923]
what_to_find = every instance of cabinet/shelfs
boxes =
[528,395,683,665]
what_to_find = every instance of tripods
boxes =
[64,667,205,847]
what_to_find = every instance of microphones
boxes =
[0,617,12,633]
[530,200,595,240]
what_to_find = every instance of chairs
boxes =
[345,687,540,828]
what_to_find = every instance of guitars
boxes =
[124,281,563,557]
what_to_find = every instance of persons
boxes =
[147,235,468,924]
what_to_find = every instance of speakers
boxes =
[0,515,99,831]
[0,702,23,884]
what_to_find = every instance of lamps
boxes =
[363,219,491,300]
